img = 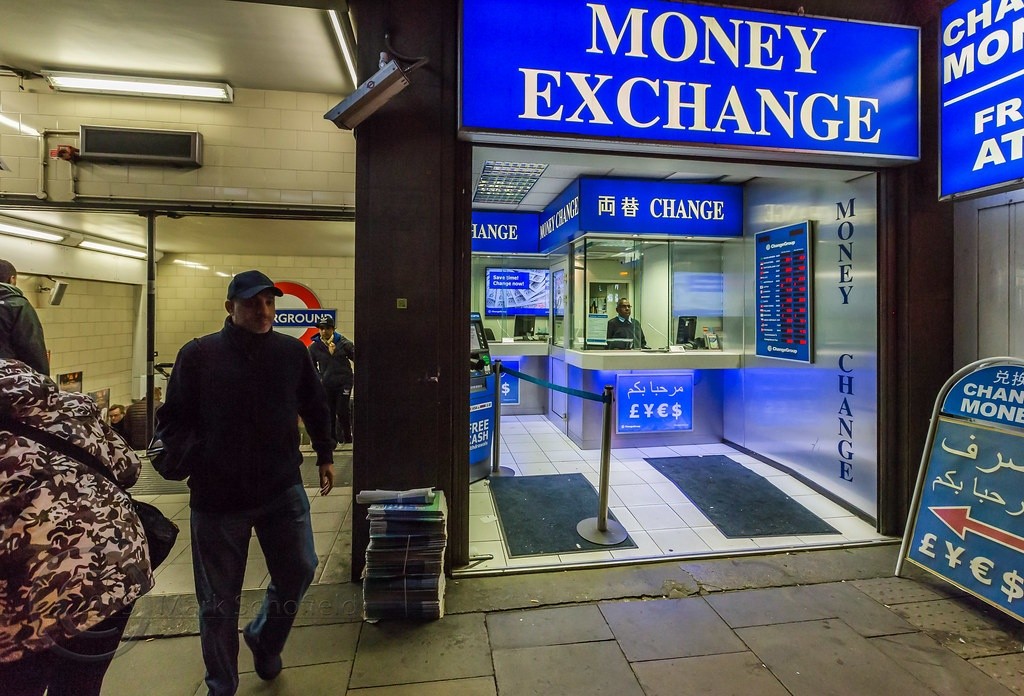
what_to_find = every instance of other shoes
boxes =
[243,621,282,680]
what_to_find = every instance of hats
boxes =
[227,270,284,301]
[314,317,335,328]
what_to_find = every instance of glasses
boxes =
[109,414,118,418]
[618,306,632,309]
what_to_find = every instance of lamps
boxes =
[38,279,69,305]
[40,69,234,104]
[324,10,358,91]
[0,215,164,264]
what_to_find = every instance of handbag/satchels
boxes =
[148,435,195,481]
[129,498,179,571]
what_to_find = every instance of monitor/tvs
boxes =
[471,322,481,352]
[514,314,535,337]
[676,315,698,344]
[484,266,550,319]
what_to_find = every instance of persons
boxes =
[0,346,156,695]
[306,317,355,444]
[0,258,51,377]
[607,297,646,350]
[123,387,165,450]
[165,270,339,696]
[109,404,126,436]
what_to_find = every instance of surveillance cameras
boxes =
[324,59,412,129]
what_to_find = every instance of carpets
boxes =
[643,454,843,540]
[485,472,639,559]
[123,454,353,497]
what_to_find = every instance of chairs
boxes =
[483,328,495,340]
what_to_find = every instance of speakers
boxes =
[48,280,69,305]
